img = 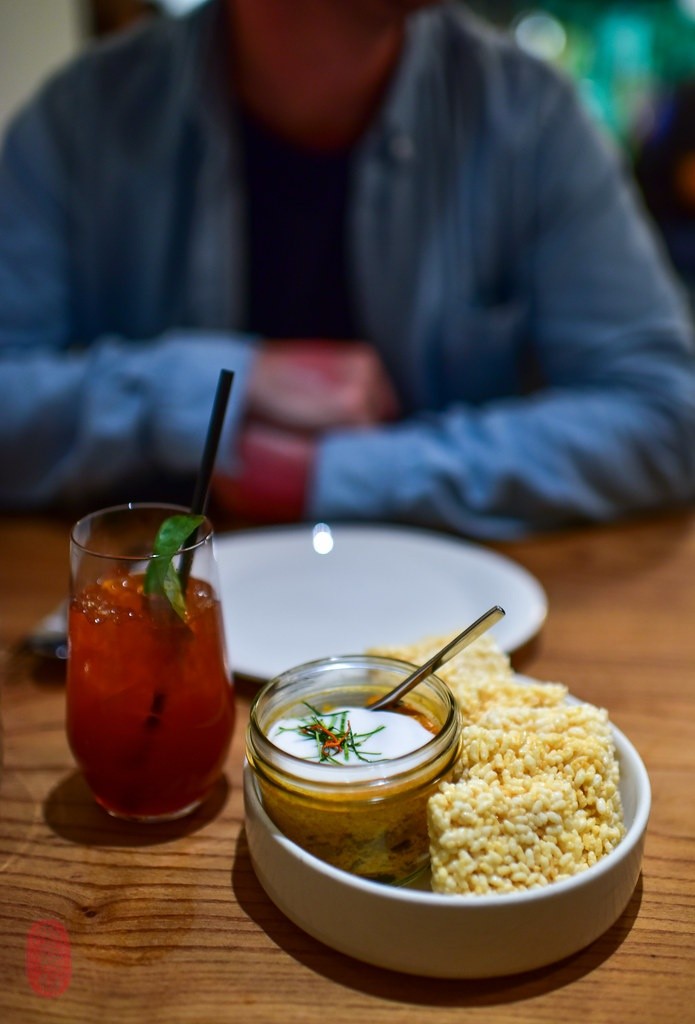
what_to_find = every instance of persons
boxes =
[1,0,695,543]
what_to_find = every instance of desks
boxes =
[0,499,695,1024]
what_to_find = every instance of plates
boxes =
[36,530,547,685]
[242,675,652,977]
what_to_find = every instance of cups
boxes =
[64,503,236,830]
[246,655,462,885]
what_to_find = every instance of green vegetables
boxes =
[271,698,391,766]
[142,512,205,638]
